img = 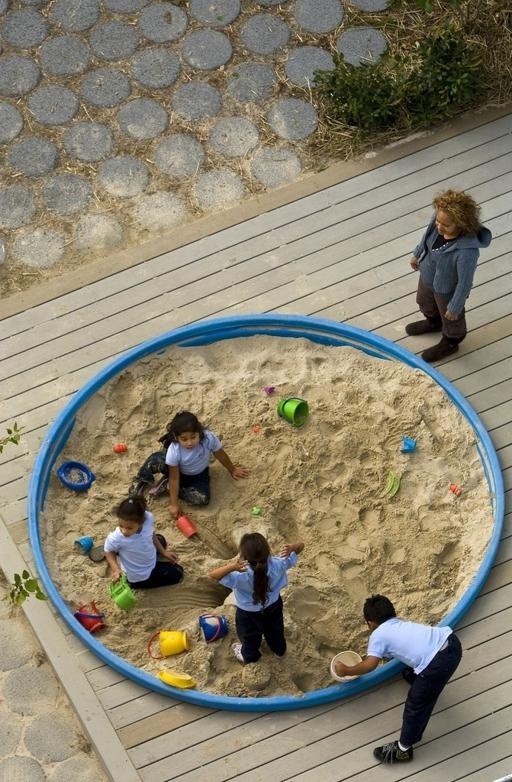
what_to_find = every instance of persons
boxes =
[336,594,462,763]
[127,411,250,519]
[104,495,182,589]
[406,188,492,363]
[208,533,304,665]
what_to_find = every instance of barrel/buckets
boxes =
[146,625,192,660]
[73,599,106,635]
[56,459,97,491]
[328,649,365,683]
[194,613,229,645]
[276,394,310,427]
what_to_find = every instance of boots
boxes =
[405,313,443,337]
[422,335,462,363]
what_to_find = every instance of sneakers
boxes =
[229,643,244,662]
[403,668,417,681]
[374,742,414,762]
[127,476,145,497]
[148,476,169,497]
